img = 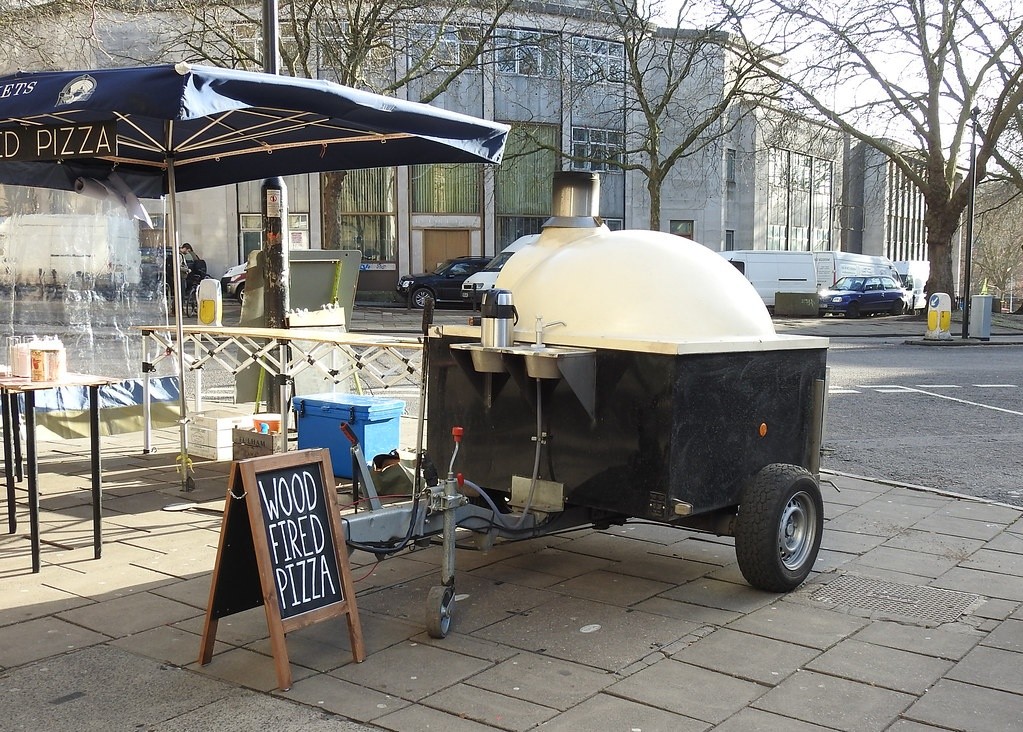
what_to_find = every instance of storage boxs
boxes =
[292,392,406,480]
[231,425,298,460]
[187,408,252,460]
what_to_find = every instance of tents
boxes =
[0,63,510,493]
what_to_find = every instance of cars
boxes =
[220,261,248,305]
[139,247,207,296]
[818,276,907,319]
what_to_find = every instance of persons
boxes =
[171,243,193,316]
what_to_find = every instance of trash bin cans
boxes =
[970,294,994,341]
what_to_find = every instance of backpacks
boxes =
[166,253,185,281]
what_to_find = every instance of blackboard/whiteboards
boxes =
[199,447,366,633]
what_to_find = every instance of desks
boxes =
[0,372,124,574]
[129,325,423,455]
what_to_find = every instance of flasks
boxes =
[479,288,518,348]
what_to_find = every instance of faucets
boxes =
[530,315,567,348]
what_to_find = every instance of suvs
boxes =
[395,255,495,309]
[1004,294,1018,303]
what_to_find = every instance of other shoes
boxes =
[170,309,175,317]
[182,311,188,317]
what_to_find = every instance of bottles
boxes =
[5,333,67,383]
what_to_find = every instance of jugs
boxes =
[196,279,224,327]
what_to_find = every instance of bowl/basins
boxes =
[253,414,281,433]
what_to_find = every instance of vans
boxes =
[460,232,541,304]
[0,214,142,300]
[715,250,818,311]
[894,261,930,309]
[812,251,908,289]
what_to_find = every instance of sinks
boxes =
[449,343,525,373]
[503,347,597,379]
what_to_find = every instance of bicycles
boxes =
[186,267,215,318]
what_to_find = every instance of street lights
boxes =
[961,107,983,338]
